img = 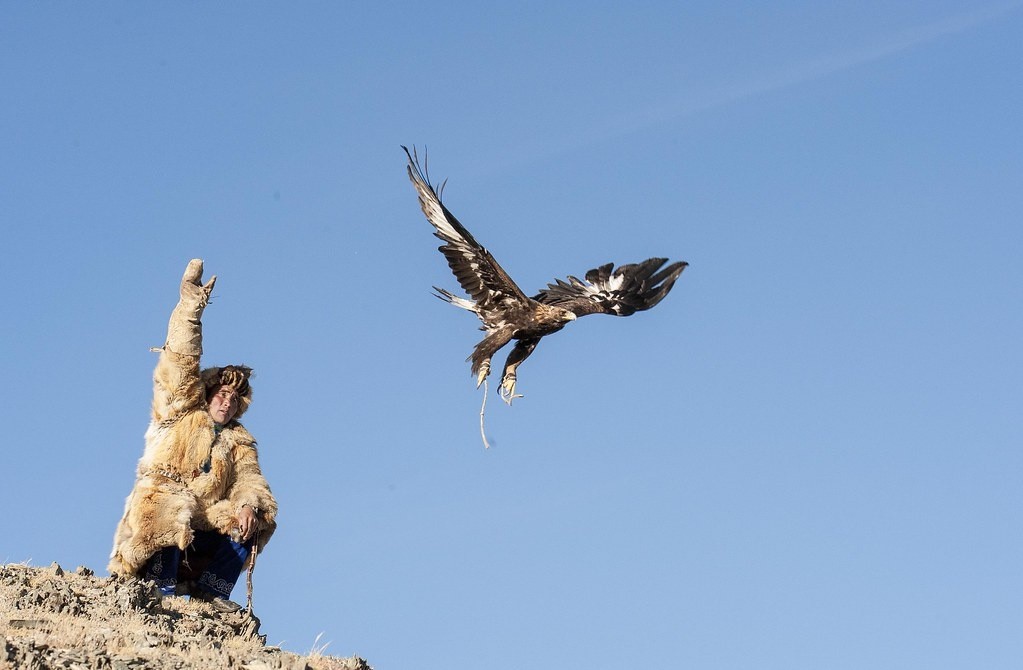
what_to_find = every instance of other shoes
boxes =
[194,589,242,613]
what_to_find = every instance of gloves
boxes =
[165,259,218,356]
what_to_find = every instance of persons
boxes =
[106,258,279,603]
